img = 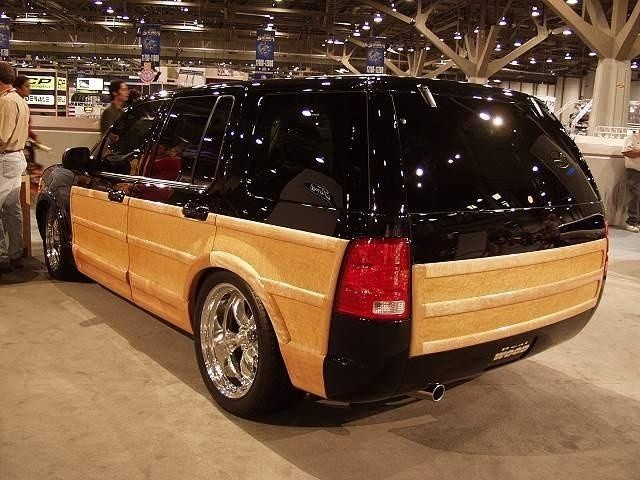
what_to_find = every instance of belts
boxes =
[0,150,20,154]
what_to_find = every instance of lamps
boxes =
[90,0,640,70]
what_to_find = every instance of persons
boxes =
[100,80,130,136]
[621,131,640,233]
[1,61,44,274]
[121,142,182,202]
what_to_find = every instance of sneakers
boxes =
[623,220,640,232]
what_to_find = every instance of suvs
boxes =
[35,72,611,422]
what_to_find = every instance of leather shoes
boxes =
[0,258,24,273]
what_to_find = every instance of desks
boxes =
[20,175,32,257]
